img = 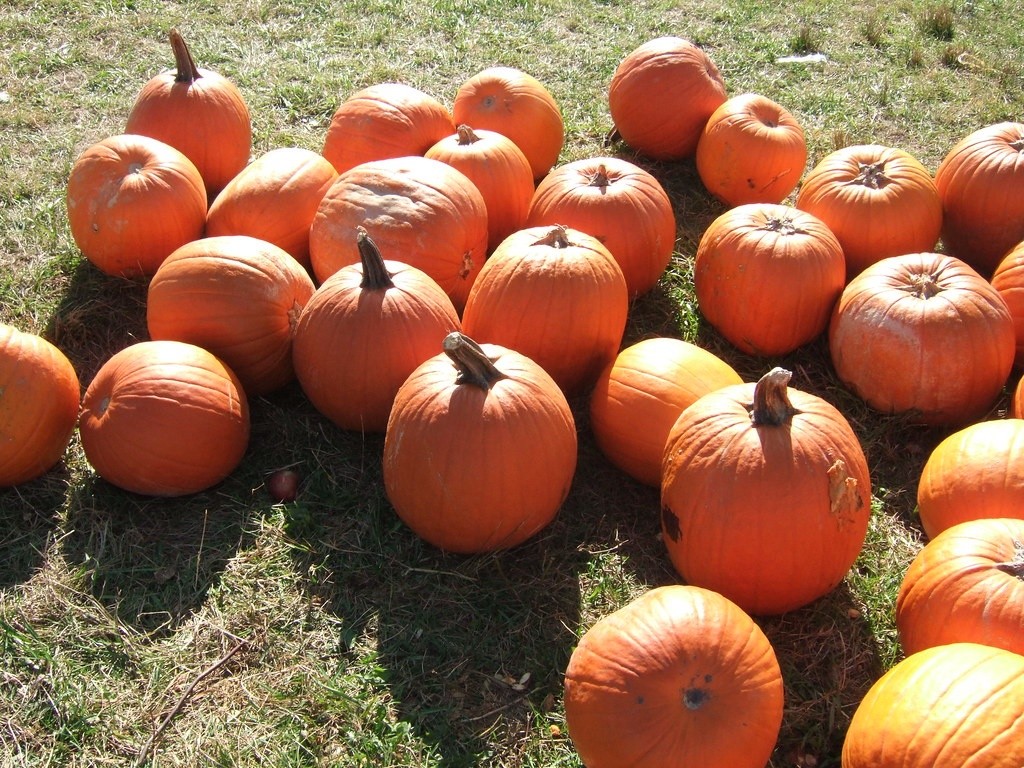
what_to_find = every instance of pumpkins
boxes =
[0,322,76,490]
[68,28,1024,768]
